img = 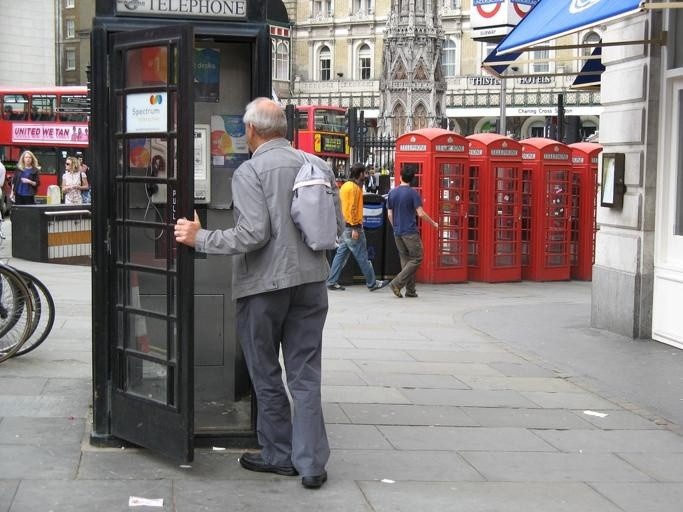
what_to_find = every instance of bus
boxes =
[292,103,352,191]
[0,82,91,219]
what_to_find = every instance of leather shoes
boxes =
[302,470,329,489]
[237,451,299,477]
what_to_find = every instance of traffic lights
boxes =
[343,105,357,146]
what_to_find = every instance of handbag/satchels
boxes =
[80,172,91,203]
[291,159,340,252]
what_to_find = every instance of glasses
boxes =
[65,162,69,165]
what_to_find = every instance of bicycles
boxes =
[0,195,55,363]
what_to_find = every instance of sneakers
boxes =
[388,282,403,298]
[327,281,345,291]
[368,279,389,291]
[0,229,6,238]
[405,289,417,297]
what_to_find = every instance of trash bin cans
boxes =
[379,175,390,194]
[327,195,400,286]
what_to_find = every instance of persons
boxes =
[60,155,89,206]
[324,163,389,293]
[383,166,440,300]
[77,161,92,203]
[11,150,43,206]
[172,94,345,489]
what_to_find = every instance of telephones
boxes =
[147,124,211,204]
